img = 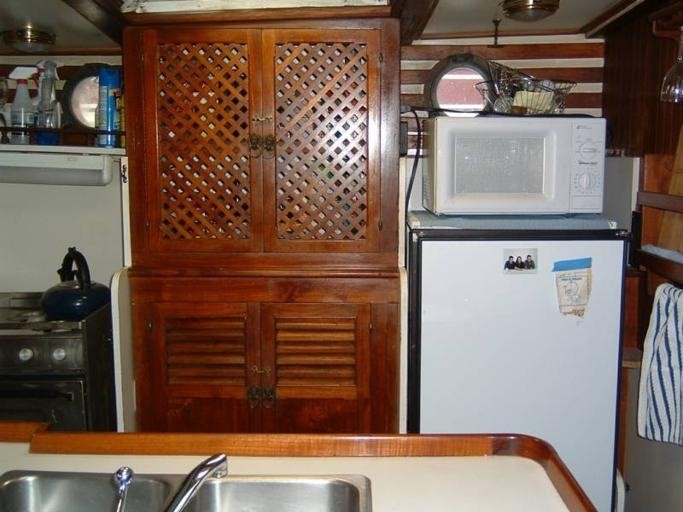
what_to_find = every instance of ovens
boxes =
[0,379,118,431]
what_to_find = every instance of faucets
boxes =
[159,452,229,512]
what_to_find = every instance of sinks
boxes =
[2,468,376,512]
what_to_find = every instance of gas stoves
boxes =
[0,288,115,377]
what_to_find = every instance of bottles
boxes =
[8,76,35,144]
[34,61,60,146]
[97,68,122,146]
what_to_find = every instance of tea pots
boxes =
[37,248,111,317]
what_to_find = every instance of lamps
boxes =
[498,0,561,23]
[3,23,56,55]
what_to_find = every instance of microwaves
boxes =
[420,114,611,218]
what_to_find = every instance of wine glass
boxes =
[654,24,682,105]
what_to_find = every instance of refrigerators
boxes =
[405,213,626,510]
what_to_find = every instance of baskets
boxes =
[472,77,577,118]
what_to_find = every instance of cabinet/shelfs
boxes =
[121,259,402,438]
[595,1,682,158]
[117,7,401,271]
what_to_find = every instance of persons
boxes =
[514,256,525,271]
[526,255,535,267]
[504,255,515,270]
